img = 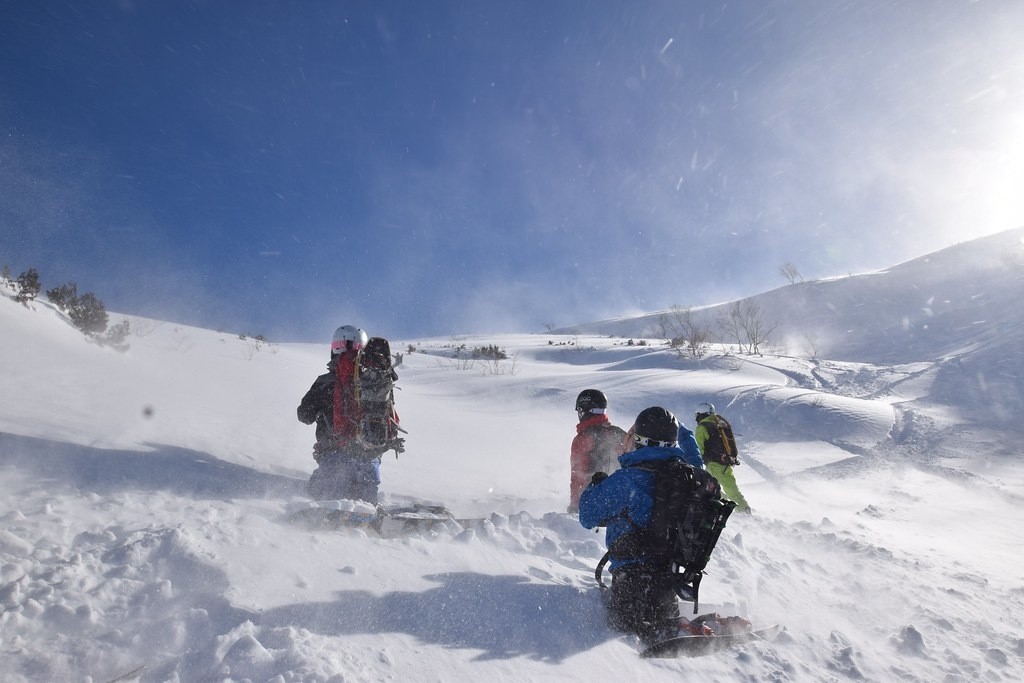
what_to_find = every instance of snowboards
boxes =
[279,504,497,539]
[632,622,788,663]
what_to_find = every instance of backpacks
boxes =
[617,460,739,575]
[699,415,738,466]
[585,423,628,478]
[333,336,405,456]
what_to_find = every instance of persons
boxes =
[567,389,628,515]
[297,326,400,528]
[694,402,751,515]
[578,407,752,647]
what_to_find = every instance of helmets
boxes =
[332,325,369,355]
[575,389,608,412]
[695,402,715,415]
[633,406,679,452]
